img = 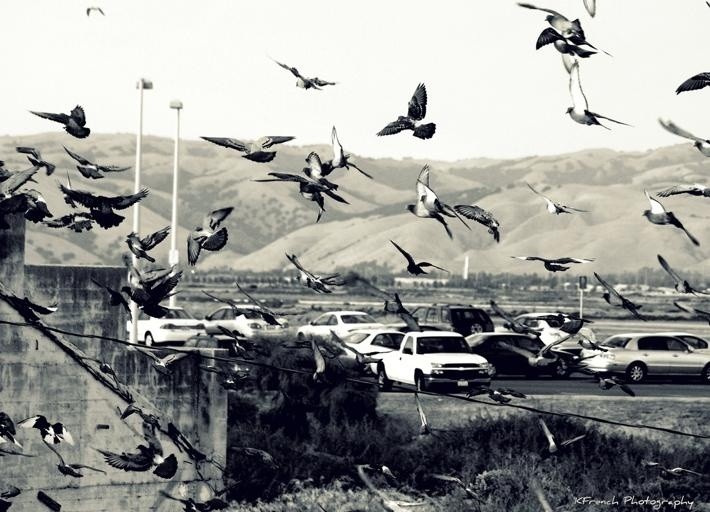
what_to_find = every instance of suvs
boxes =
[387,304,496,338]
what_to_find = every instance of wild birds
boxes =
[99,355,122,390]
[535,26,598,58]
[466,385,527,405]
[134,346,192,377]
[185,206,235,266]
[498,335,571,368]
[376,82,436,140]
[63,170,95,208]
[578,367,636,397]
[565,58,634,131]
[124,225,172,263]
[552,313,593,335]
[6,294,60,323]
[659,117,710,157]
[403,162,454,241]
[328,124,374,181]
[594,272,647,322]
[656,253,710,299]
[17,414,76,448]
[198,136,296,163]
[340,340,392,365]
[274,60,337,91]
[416,177,472,232]
[87,6,104,17]
[200,289,261,320]
[526,183,592,216]
[389,239,450,276]
[89,277,133,322]
[216,324,263,361]
[489,299,544,337]
[509,255,597,272]
[121,263,185,320]
[537,414,586,457]
[42,439,106,478]
[284,252,350,294]
[252,171,351,223]
[454,203,500,244]
[96,432,179,480]
[414,391,452,441]
[516,2,598,51]
[383,292,424,333]
[235,281,309,328]
[16,146,56,176]
[202,314,240,343]
[279,338,340,384]
[640,458,702,482]
[120,402,162,431]
[41,211,95,233]
[300,150,339,192]
[62,145,133,179]
[641,187,700,246]
[576,334,616,352]
[28,105,90,139]
[57,180,151,230]
[655,182,710,199]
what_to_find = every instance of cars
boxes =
[201,303,289,342]
[671,332,709,357]
[125,304,203,347]
[292,308,387,346]
[377,323,444,334]
[465,331,576,380]
[580,332,710,384]
[334,329,405,365]
[495,313,597,352]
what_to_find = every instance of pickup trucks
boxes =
[367,331,495,396]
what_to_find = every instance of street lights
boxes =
[128,76,156,343]
[166,98,184,307]
